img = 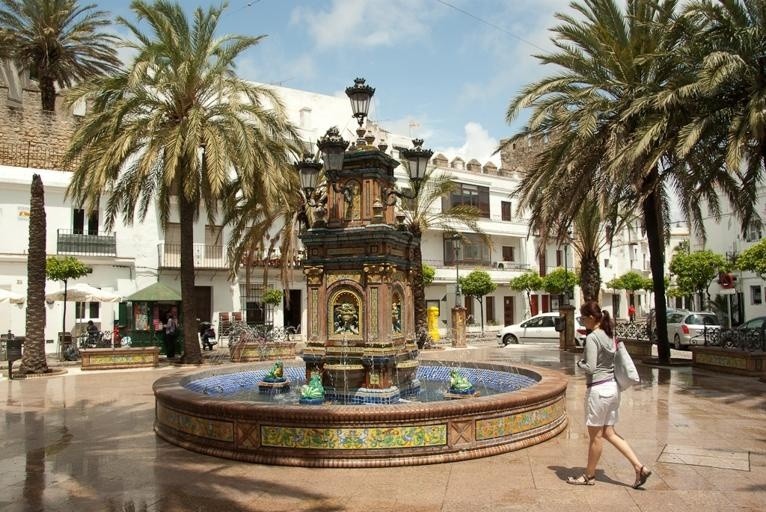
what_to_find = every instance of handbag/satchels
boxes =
[166,325,176,337]
[614,341,640,391]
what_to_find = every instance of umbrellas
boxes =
[46,283,125,348]
[127,281,182,305]
[0,289,25,306]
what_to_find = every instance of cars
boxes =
[720,317,765,353]
[497,311,589,348]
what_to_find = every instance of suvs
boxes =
[654,308,723,349]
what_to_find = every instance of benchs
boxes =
[611,321,652,355]
[690,327,766,376]
[77,331,162,370]
[229,324,300,361]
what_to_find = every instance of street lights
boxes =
[558,230,575,350]
[451,231,467,349]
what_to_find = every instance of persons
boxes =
[87,320,98,344]
[566,302,651,489]
[629,304,636,322]
[163,313,177,358]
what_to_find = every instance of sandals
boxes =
[568,472,595,485]
[634,465,651,489]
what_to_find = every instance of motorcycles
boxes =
[196,318,217,350]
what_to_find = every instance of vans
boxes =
[646,309,674,344]
